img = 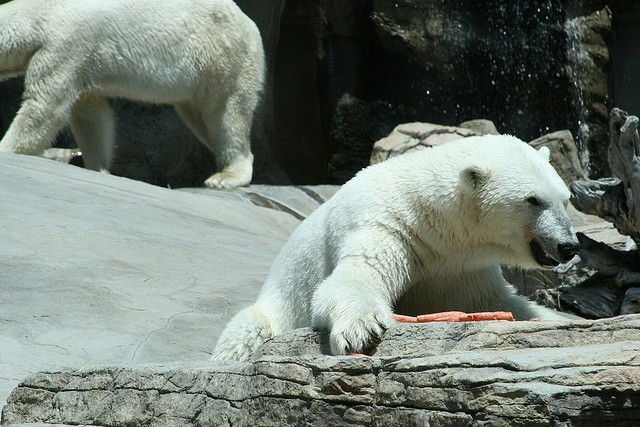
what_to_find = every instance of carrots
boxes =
[396,314,418,322]
[458,312,514,320]
[417,311,466,322]
